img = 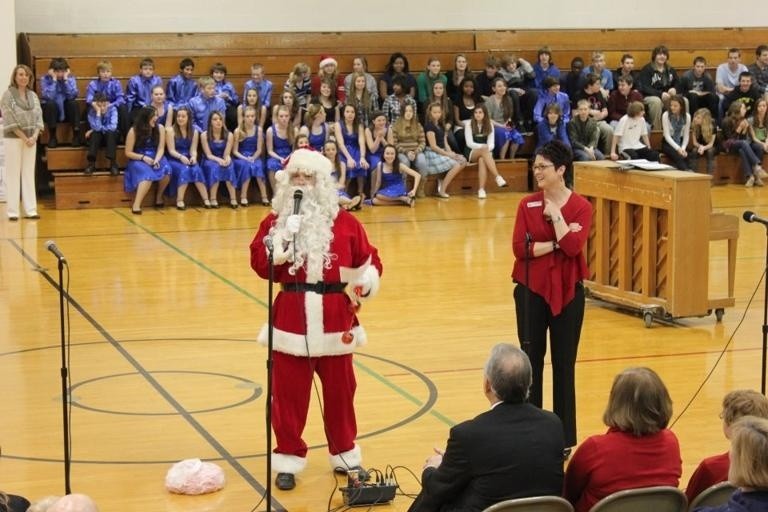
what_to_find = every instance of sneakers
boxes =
[744,165,768,188]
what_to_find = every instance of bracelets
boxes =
[552,215,564,225]
[552,239,557,251]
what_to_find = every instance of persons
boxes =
[693,415,768,511]
[560,367,681,511]
[510,140,594,462]
[685,390,768,508]
[44,492,96,512]
[1,65,45,220]
[407,342,564,511]
[248,148,383,490]
[39,57,81,148]
[0,491,30,512]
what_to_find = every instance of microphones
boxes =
[293,190,303,215]
[44,239,65,264]
[741,210,768,228]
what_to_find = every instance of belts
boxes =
[279,280,349,293]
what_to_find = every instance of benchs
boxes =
[19,26,768,215]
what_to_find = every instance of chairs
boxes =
[588,484,689,511]
[689,480,738,512]
[480,495,574,511]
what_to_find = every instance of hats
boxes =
[318,54,338,68]
[279,144,333,172]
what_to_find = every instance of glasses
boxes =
[532,164,561,172]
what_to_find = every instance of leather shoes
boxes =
[176,197,186,211]
[8,215,41,220]
[335,464,373,481]
[201,198,212,208]
[339,175,507,212]
[229,199,239,208]
[260,196,270,206]
[131,203,141,214]
[210,198,219,208]
[240,195,249,207]
[154,189,164,208]
[70,136,81,147]
[109,164,120,176]
[84,164,97,173]
[275,472,296,490]
[47,137,58,148]
